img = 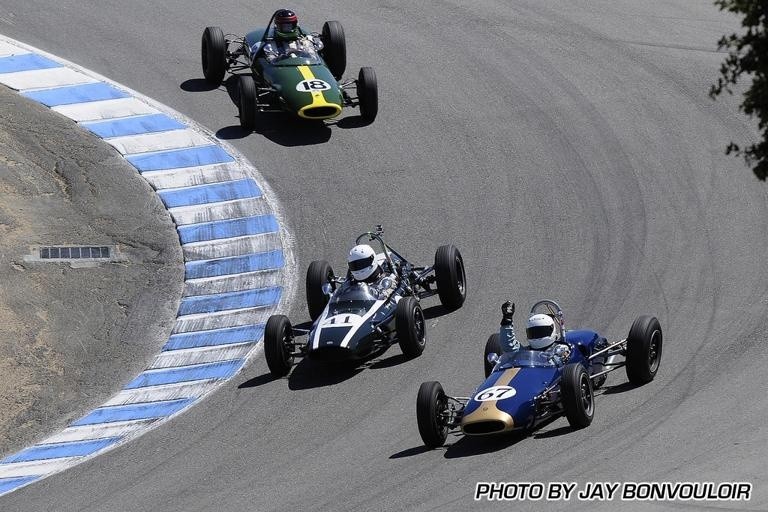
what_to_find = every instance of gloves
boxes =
[500,300,516,326]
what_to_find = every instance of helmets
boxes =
[348,244,379,281]
[274,9,298,32]
[525,313,556,349]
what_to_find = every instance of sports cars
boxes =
[264,224,663,447]
[201,8,378,128]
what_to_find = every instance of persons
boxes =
[509,313,571,369]
[349,244,395,298]
[263,8,312,64]
